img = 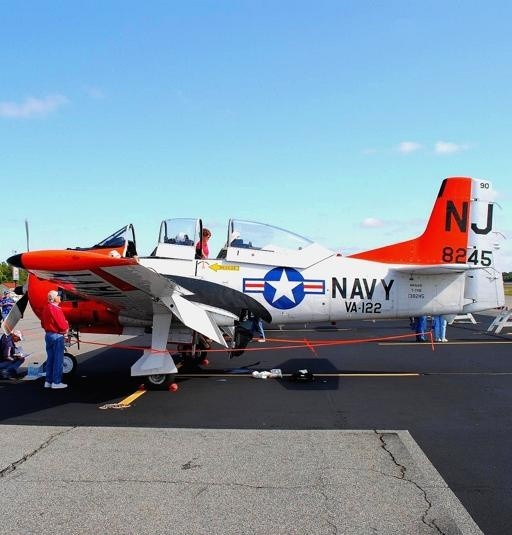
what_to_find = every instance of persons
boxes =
[414,314,429,342]
[195,227,212,260]
[0,327,27,383]
[432,315,449,343]
[40,289,71,391]
[1,289,17,322]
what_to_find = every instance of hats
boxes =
[48,289,62,299]
[4,289,9,294]
[14,330,23,342]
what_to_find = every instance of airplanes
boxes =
[1,177,505,392]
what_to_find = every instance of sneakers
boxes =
[44,382,68,389]
